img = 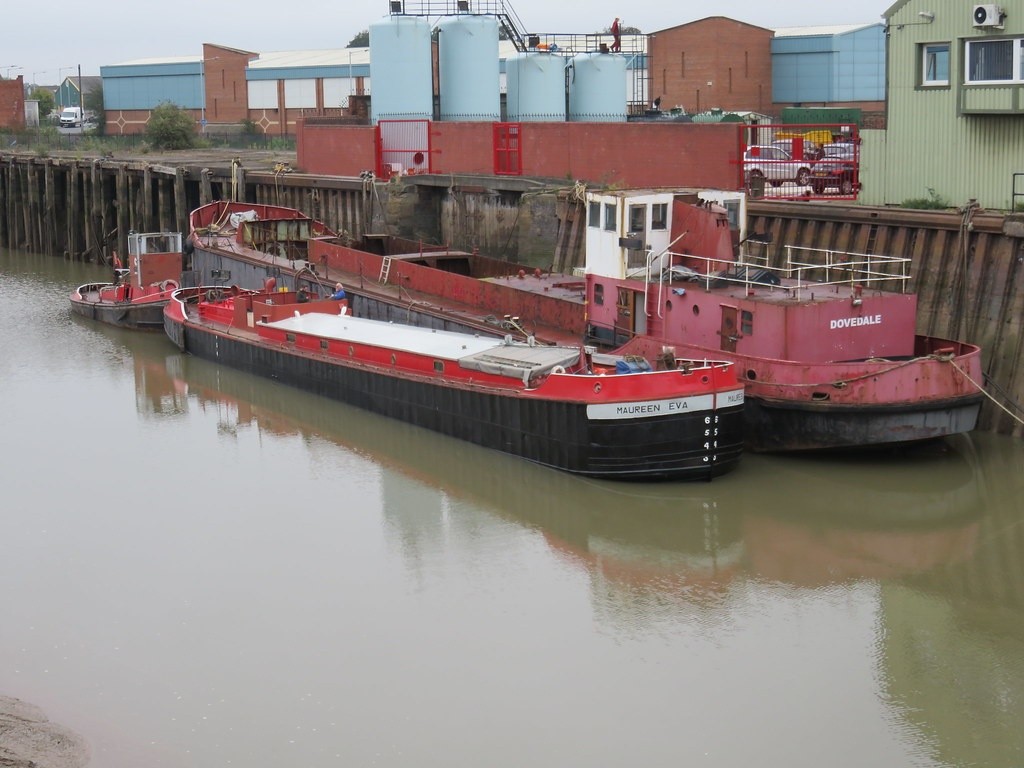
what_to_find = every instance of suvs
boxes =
[744,145,811,186]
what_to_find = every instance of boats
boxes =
[165,186,988,482]
[69,230,185,332]
[162,282,758,483]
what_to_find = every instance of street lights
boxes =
[58,66,72,110]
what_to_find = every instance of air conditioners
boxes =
[972,4,1005,30]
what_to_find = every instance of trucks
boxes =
[59,106,86,128]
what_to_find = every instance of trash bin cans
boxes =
[750,176,766,199]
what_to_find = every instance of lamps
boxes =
[918,11,935,20]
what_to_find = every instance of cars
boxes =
[772,140,821,163]
[817,143,860,162]
[807,155,858,194]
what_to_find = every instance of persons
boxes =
[655,96,661,110]
[329,282,345,300]
[610,18,619,52]
[296,283,310,303]
[815,143,825,161]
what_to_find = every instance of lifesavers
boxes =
[550,366,567,375]
[160,279,179,291]
